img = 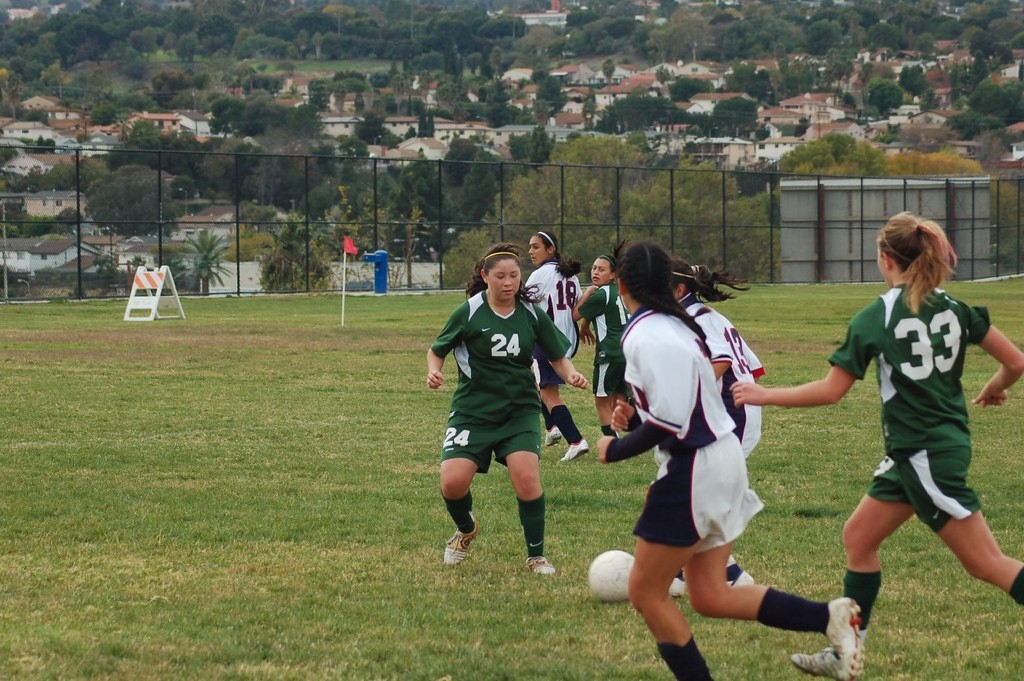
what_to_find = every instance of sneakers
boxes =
[443,519,478,565]
[544,425,562,447]
[521,555,555,574]
[827,598,865,681]
[791,647,843,680]
[558,438,590,462]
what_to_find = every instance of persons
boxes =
[596,240,865,681]
[524,231,766,598]
[729,212,1024,678]
[426,245,590,575]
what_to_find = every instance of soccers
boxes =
[588,549,635,604]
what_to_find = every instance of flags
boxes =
[344,235,358,254]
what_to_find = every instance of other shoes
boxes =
[726,570,754,588]
[668,578,686,597]
